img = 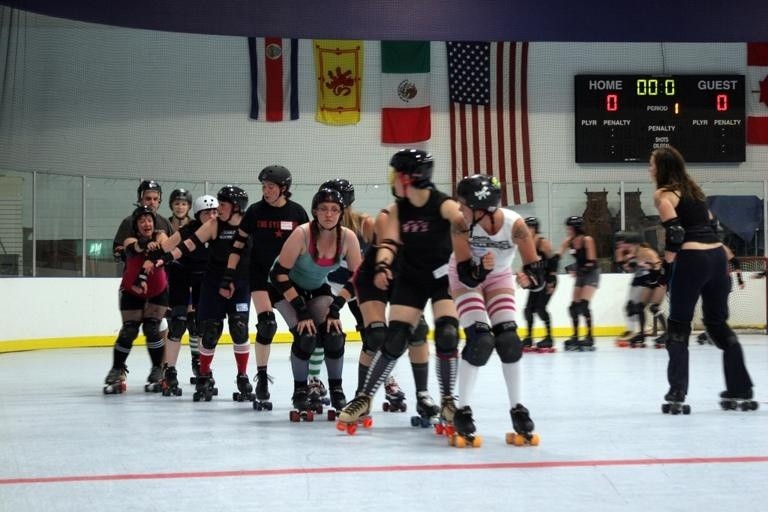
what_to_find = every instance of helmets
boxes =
[614,231,642,242]
[258,165,292,192]
[168,188,193,211]
[566,216,586,234]
[389,148,434,181]
[217,186,248,210]
[193,195,220,219]
[132,206,156,234]
[138,179,162,204]
[525,216,539,228]
[312,188,345,212]
[458,173,501,209]
[319,179,355,209]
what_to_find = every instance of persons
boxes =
[349,164,439,420]
[102,180,256,403]
[219,165,310,410]
[266,180,439,428]
[446,174,547,436]
[332,149,464,422]
[647,148,756,401]
[509,215,768,354]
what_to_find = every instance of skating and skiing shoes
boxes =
[521,335,557,353]
[505,403,540,446]
[662,390,690,414]
[103,362,273,411]
[565,334,596,352]
[697,332,713,344]
[290,377,481,447]
[718,385,758,411]
[614,329,666,348]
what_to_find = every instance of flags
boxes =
[445,41,532,208]
[744,42,767,146]
[312,38,363,126]
[248,38,301,122]
[379,42,430,145]
